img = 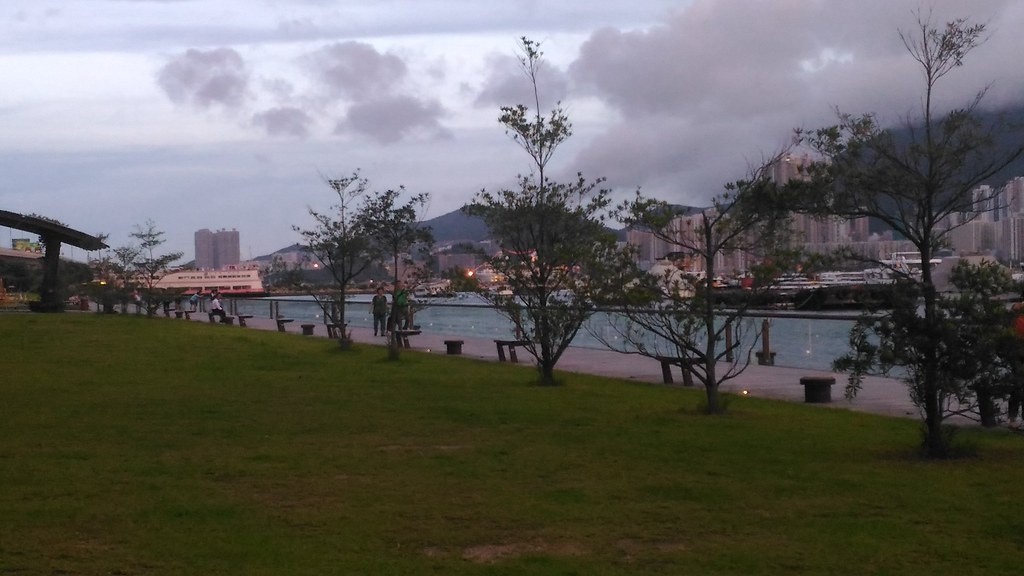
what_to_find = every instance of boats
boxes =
[862,251,941,283]
[488,284,513,301]
[413,277,453,297]
[702,271,828,306]
[111,265,270,297]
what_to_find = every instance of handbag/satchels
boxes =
[387,318,392,331]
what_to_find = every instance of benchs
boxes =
[494,340,532,363]
[185,311,196,319]
[656,356,717,387]
[969,383,1015,428]
[209,313,220,323]
[164,309,175,317]
[326,323,347,339]
[395,331,422,349]
[277,320,295,332]
[239,316,253,327]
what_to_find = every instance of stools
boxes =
[800,377,836,403]
[301,325,315,335]
[756,352,776,366]
[175,312,183,318]
[224,317,235,325]
[403,326,421,330]
[444,340,465,355]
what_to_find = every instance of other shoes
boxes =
[381,335,385,336]
[375,333,376,336]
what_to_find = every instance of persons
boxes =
[1006,300,1024,431]
[134,291,142,313]
[69,295,80,303]
[369,287,387,336]
[190,290,204,311]
[397,280,409,330]
[212,293,226,323]
